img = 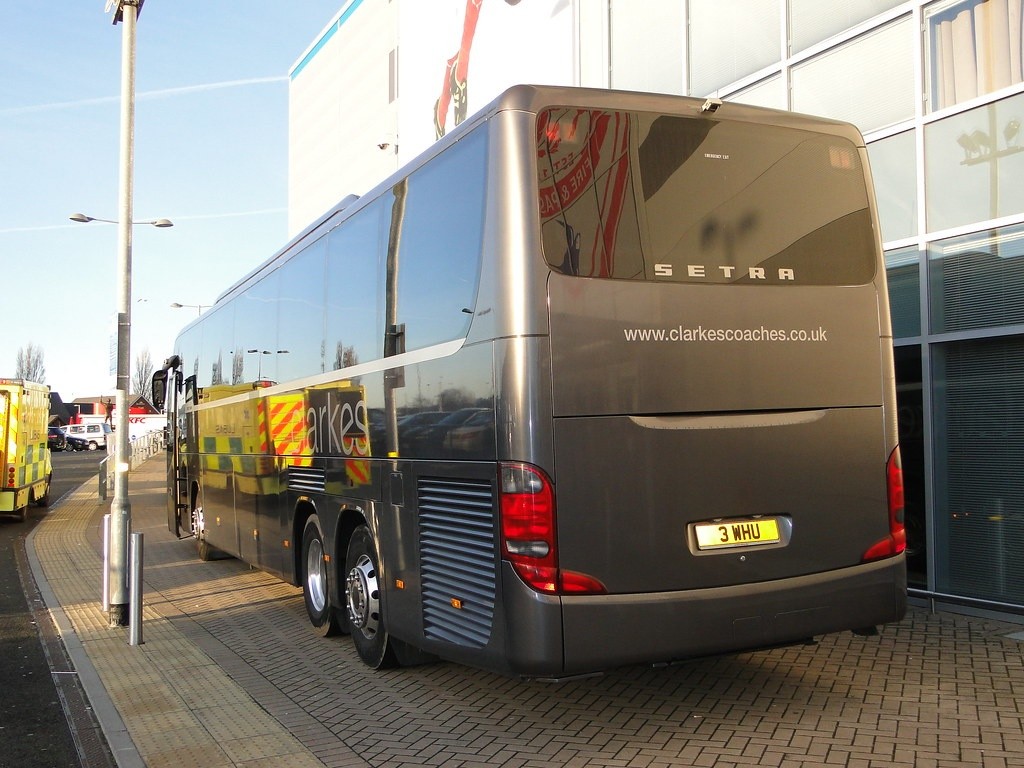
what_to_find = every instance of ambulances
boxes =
[1,377,53,520]
[181,379,372,497]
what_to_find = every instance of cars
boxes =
[66,434,89,452]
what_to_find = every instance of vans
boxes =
[60,423,111,450]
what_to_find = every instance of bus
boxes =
[152,82,910,674]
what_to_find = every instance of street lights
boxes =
[171,301,212,319]
[248,349,270,379]
[69,1,176,621]
[74,405,80,414]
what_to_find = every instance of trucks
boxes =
[77,415,168,445]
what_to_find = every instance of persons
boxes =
[99,395,113,432]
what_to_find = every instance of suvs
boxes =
[48,427,67,452]
